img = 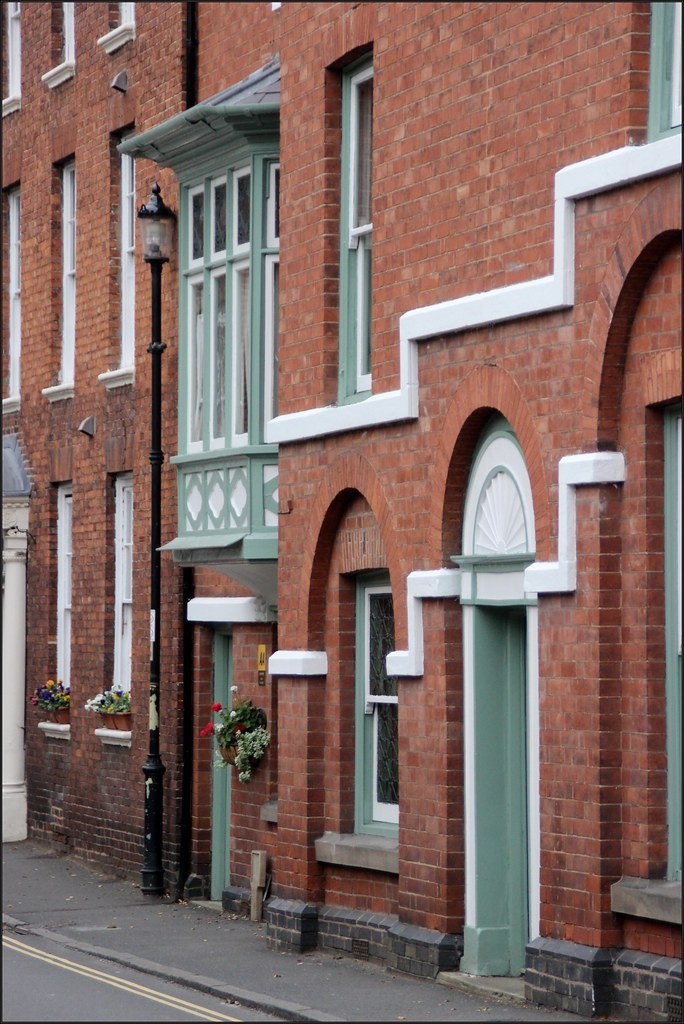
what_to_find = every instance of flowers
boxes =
[200,686,270,785]
[29,679,70,711]
[84,685,131,712]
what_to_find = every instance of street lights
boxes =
[134,181,178,896]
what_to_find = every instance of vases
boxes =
[55,707,70,724]
[100,712,117,730]
[45,709,58,723]
[219,744,239,763]
[110,712,132,731]
